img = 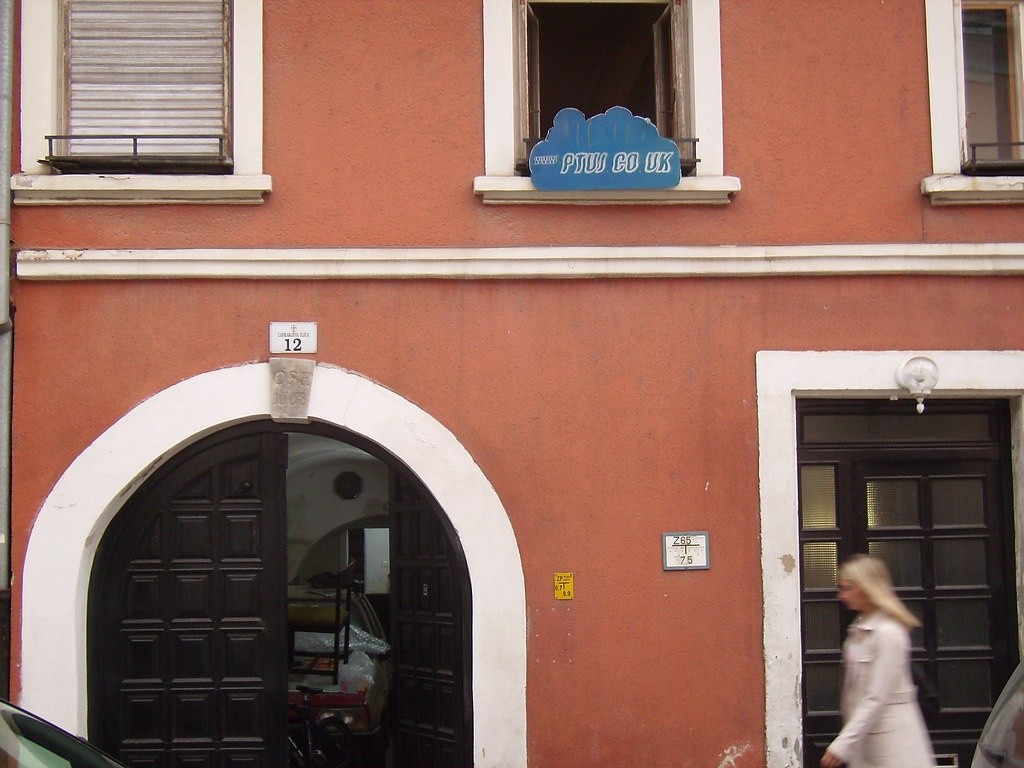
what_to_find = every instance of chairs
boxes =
[287,558,357,686]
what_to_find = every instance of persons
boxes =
[821,555,935,768]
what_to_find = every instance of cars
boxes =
[286,583,394,768]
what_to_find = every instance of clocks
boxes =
[334,471,362,499]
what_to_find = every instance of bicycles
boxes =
[287,660,363,767]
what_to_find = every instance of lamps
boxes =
[897,354,941,415]
